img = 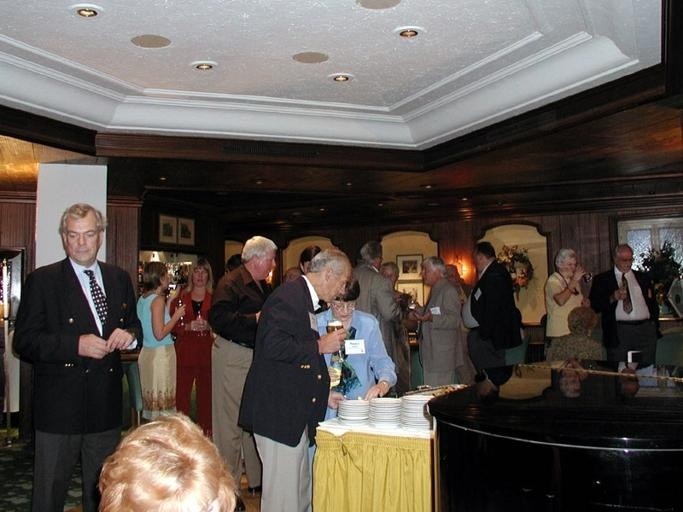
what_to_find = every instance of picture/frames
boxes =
[158,214,178,245]
[177,216,195,249]
[395,253,424,282]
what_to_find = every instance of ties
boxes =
[83,269,107,324]
[621,273,632,315]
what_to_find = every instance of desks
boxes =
[309,382,467,512]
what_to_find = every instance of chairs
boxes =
[655,333,683,377]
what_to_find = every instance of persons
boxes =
[5,205,142,510]
[546,306,606,362]
[592,244,663,367]
[240,247,352,512]
[462,239,523,376]
[544,247,584,358]
[98,413,246,512]
[127,242,468,440]
[469,363,646,399]
[211,235,263,511]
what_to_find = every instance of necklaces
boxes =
[556,270,580,296]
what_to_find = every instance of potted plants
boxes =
[636,239,681,315]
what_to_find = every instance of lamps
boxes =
[449,253,464,277]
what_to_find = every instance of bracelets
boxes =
[564,287,573,295]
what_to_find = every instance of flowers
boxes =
[494,243,534,301]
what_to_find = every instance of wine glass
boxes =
[196,310,207,337]
[176,298,186,326]
[571,266,593,282]
[326,319,349,363]
[407,298,418,310]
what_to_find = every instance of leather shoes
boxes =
[235,499,246,512]
[248,485,262,494]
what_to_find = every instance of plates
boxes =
[338,395,435,432]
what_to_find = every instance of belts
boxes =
[221,335,253,350]
